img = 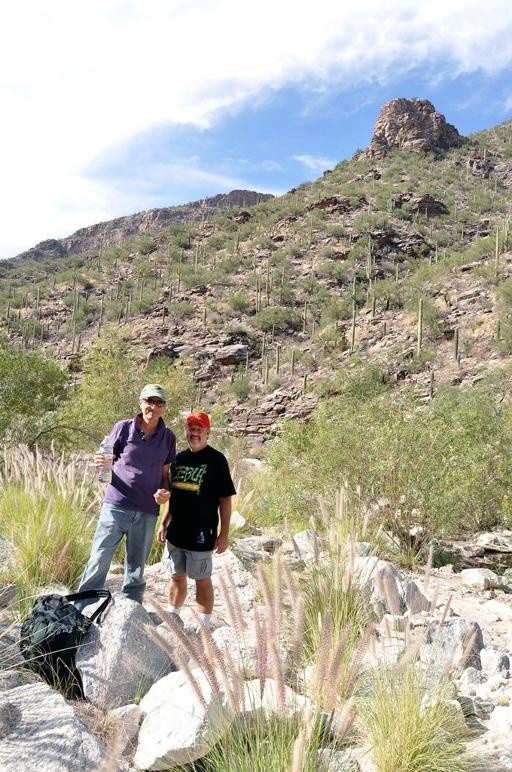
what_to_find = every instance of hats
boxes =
[184,412,209,428]
[139,384,169,402]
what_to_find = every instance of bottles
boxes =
[98,434,114,485]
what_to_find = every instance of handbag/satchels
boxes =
[21,594,90,681]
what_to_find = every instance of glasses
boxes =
[144,398,164,407]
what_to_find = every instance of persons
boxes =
[74,384,176,615]
[156,412,237,634]
